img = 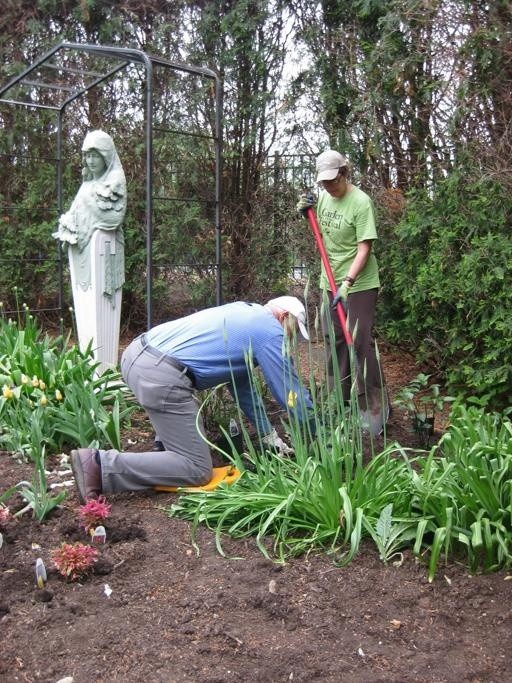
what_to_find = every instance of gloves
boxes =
[296,194,315,219]
[258,427,296,460]
[332,281,351,315]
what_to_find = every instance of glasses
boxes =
[322,173,344,188]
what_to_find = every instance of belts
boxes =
[141,334,198,388]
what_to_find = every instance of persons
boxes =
[70,294,324,506]
[295,149,393,416]
[53,130,128,380]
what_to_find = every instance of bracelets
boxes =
[345,275,355,286]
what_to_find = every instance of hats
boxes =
[315,150,347,184]
[268,296,310,341]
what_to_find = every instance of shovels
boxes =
[298,187,391,435]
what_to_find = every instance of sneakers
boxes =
[70,447,100,507]
